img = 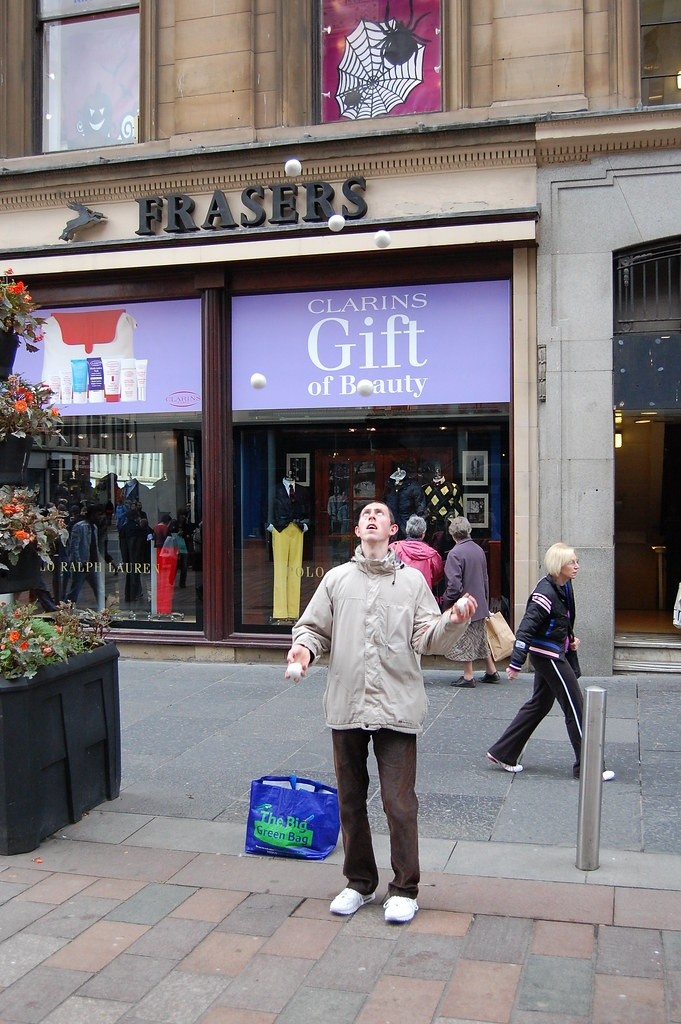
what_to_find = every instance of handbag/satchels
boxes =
[245,775,339,860]
[485,611,516,661]
[565,647,581,679]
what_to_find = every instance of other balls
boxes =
[328,215,346,232]
[456,597,468,617]
[374,230,391,248]
[287,663,302,680]
[285,159,303,177]
[250,372,266,389]
[356,379,374,395]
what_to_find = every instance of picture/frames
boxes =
[462,451,488,486]
[286,453,310,486]
[463,493,488,529]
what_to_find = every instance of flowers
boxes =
[0,268,118,679]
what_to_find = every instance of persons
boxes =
[328,486,350,559]
[387,514,444,591]
[471,456,480,480]
[382,471,463,546]
[265,475,313,621]
[284,497,478,923]
[486,544,615,781]
[443,516,501,688]
[32,498,203,614]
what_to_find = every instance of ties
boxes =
[289,485,294,500]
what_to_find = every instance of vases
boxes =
[0,642,121,856]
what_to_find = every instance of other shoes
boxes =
[486,752,523,772]
[603,771,614,780]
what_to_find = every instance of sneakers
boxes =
[330,887,375,914]
[383,895,418,921]
[451,676,476,687]
[478,672,500,682]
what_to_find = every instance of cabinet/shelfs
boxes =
[314,448,453,596]
